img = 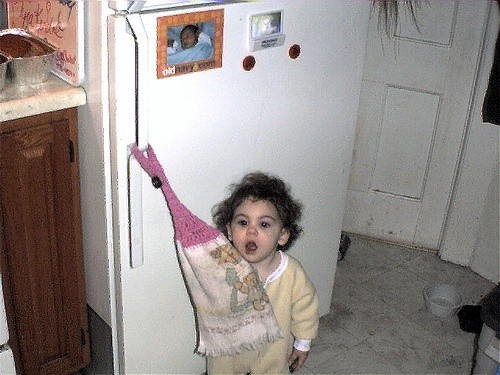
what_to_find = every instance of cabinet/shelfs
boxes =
[1,106,96,372]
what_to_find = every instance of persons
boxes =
[179,25,199,50]
[202,172,321,375]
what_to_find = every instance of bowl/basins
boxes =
[0,50,13,92]
[0,31,59,86]
[423,283,463,318]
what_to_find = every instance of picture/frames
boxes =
[156,19,224,78]
